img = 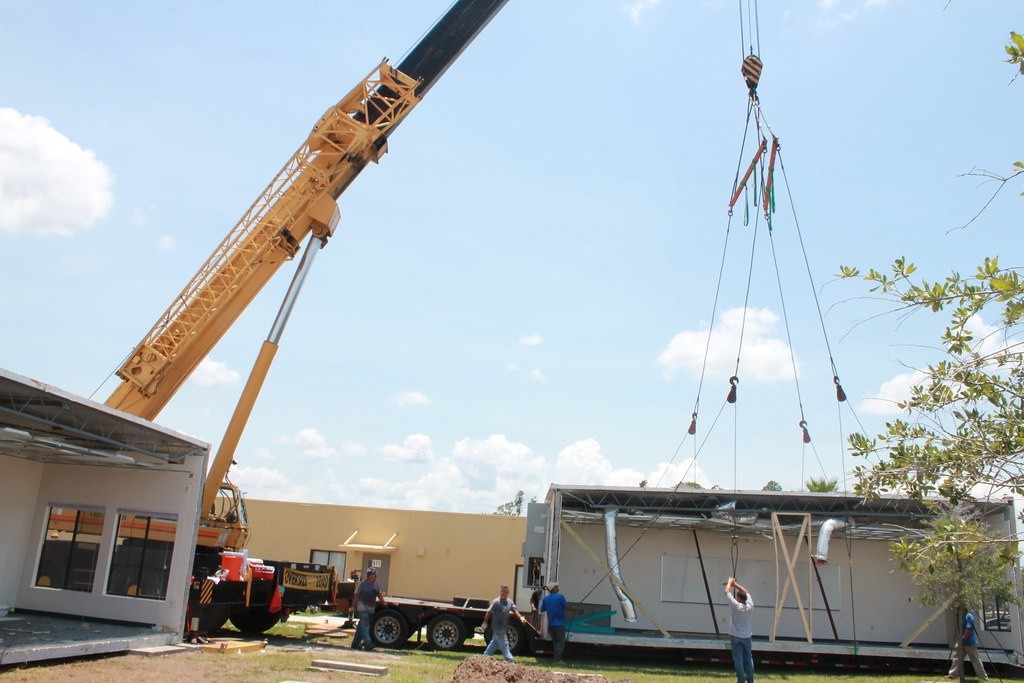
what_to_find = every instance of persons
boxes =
[724,576,754,683]
[349,567,387,650]
[944,606,988,681]
[540,582,577,664]
[530,590,542,625]
[481,585,526,661]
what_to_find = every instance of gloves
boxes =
[481,623,487,630]
[349,607,355,614]
[520,617,526,625]
[383,603,388,608]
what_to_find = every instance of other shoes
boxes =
[351,644,362,650]
[364,644,376,651]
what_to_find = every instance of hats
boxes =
[367,568,376,573]
[548,582,559,591]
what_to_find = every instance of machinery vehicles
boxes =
[29,0,517,634]
